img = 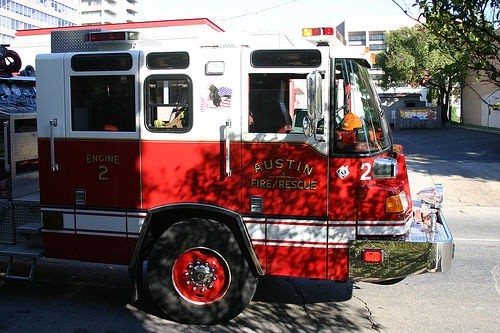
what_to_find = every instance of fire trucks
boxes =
[0,26,456,324]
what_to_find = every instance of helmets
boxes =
[340,113,362,131]
